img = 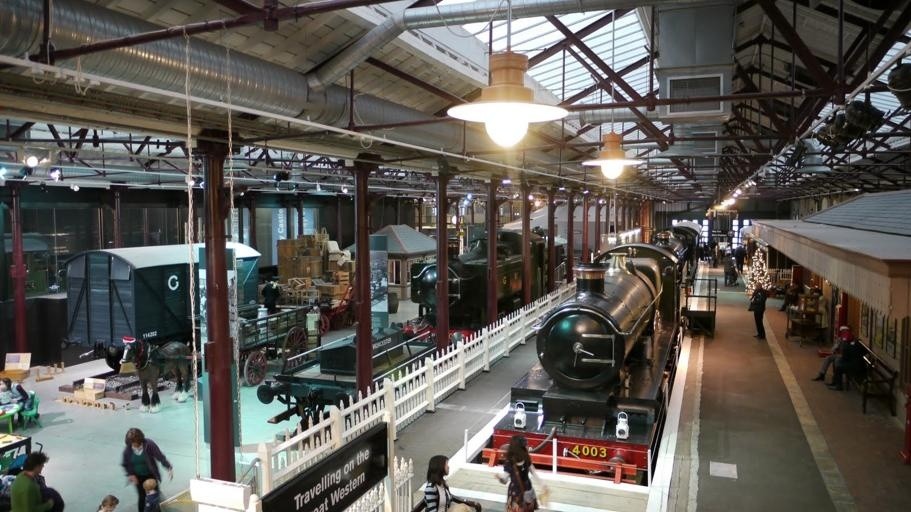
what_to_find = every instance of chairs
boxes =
[21,398,42,430]
[23,390,36,426]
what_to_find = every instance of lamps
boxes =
[447,1,571,150]
[580,1,644,181]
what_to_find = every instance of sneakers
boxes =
[812,372,844,391]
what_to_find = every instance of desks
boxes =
[1,402,20,433]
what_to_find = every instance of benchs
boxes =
[845,336,898,417]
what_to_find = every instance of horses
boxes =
[118,334,194,414]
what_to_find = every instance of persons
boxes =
[96,495,120,512]
[424,454,481,512]
[779,279,804,313]
[496,435,549,512]
[703,241,748,287]
[813,288,828,342]
[812,326,867,390]
[0,377,30,427]
[10,451,55,512]
[122,427,174,512]
[262,275,282,313]
[142,479,164,512]
[748,282,767,339]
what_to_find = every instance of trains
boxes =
[55,229,355,381]
[390,227,570,352]
[468,218,704,488]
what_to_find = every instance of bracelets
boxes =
[463,499,466,504]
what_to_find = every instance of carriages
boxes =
[119,299,309,413]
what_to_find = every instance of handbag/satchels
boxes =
[521,487,539,512]
